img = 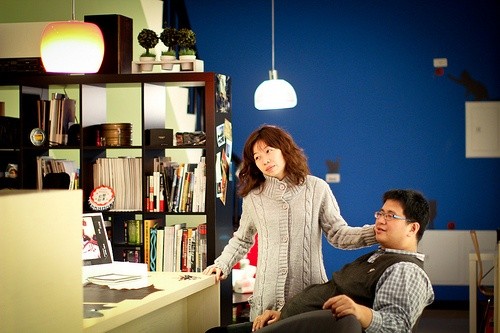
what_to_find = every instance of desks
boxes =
[468,249,494,333]
[81,272,220,333]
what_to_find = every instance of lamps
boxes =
[253,0,299,111]
[40,0,105,73]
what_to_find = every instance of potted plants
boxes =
[159,26,178,70]
[138,27,158,71]
[179,28,198,71]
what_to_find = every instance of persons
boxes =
[202,125,379,322]
[241,188,435,333]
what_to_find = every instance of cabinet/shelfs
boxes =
[0,73,235,323]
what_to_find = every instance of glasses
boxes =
[375,211,417,222]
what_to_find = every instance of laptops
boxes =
[82,213,141,282]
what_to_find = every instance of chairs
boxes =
[255,309,364,333]
[469,229,494,333]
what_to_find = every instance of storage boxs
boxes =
[146,126,173,147]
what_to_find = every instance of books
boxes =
[36,156,80,190]
[92,157,143,212]
[146,156,207,213]
[122,215,207,272]
